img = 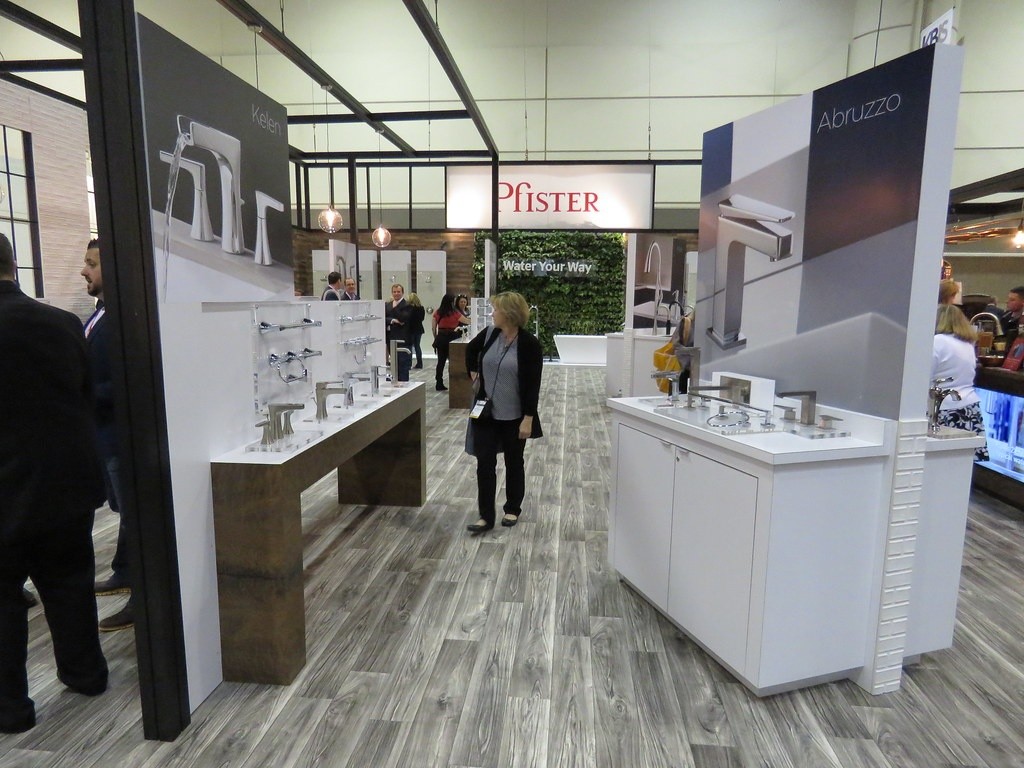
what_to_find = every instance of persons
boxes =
[668,309,695,395]
[930,304,992,462]
[23,586,37,608]
[77,237,140,632]
[463,288,543,533]
[340,277,361,302]
[982,286,1024,370]
[430,294,470,391]
[939,280,958,305]
[321,271,342,300]
[455,294,471,334]
[0,230,110,734]
[383,284,426,382]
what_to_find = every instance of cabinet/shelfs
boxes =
[610,410,884,699]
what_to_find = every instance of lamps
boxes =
[372,130,391,247]
[318,85,343,233]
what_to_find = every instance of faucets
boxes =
[669,289,696,322]
[657,306,672,337]
[526,305,540,340]
[370,365,393,396]
[343,371,370,406]
[969,310,1005,337]
[774,390,845,438]
[255,402,305,444]
[706,193,795,351]
[462,326,469,336]
[310,380,347,419]
[388,340,411,384]
[680,347,702,396]
[650,371,680,402]
[454,326,466,340]
[926,377,963,433]
[644,240,664,335]
[159,114,285,267]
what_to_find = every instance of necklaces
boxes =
[502,336,510,349]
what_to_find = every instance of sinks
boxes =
[696,312,923,423]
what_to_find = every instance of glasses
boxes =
[458,294,467,298]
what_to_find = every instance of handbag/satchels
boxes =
[653,318,692,393]
[469,399,494,425]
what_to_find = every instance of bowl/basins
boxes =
[978,354,1004,368]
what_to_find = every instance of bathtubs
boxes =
[550,330,624,364]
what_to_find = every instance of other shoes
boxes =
[502,514,518,525]
[436,385,447,390]
[57,671,67,685]
[413,365,422,369]
[22,588,36,607]
[466,518,495,532]
[98,595,135,631]
[94,575,132,595]
